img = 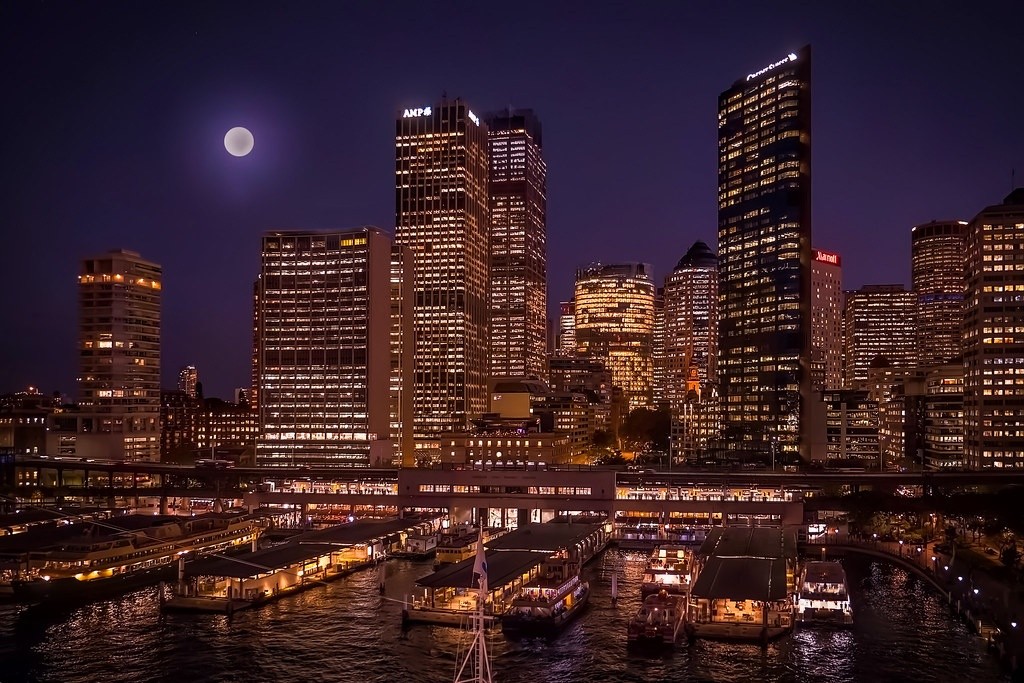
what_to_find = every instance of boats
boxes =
[390,523,505,569]
[0,515,264,587]
[160,515,432,612]
[795,558,855,627]
[628,544,692,649]
[687,524,800,635]
[499,568,594,629]
[405,523,612,625]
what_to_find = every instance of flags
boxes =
[473,534,487,593]
